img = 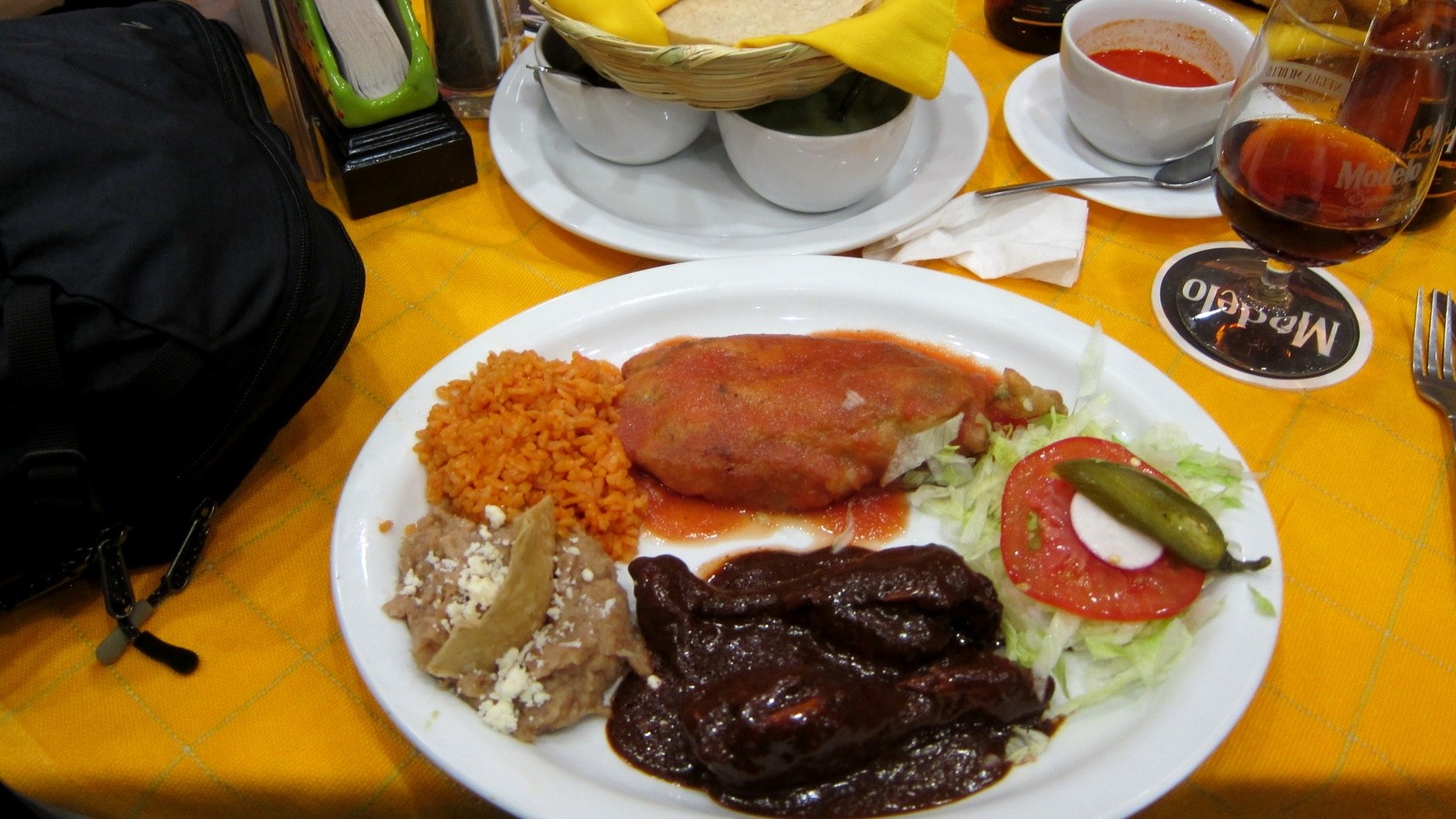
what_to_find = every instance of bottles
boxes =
[1337,1,1456,238]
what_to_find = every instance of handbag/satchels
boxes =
[5,2,364,624]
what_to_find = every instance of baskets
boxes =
[531,2,848,106]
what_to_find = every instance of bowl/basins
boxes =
[1061,0,1271,164]
[713,70,915,212]
[535,19,721,165]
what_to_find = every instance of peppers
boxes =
[1053,458,1273,575]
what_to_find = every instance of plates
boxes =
[1001,52,1301,219]
[327,252,1284,819]
[490,37,993,264]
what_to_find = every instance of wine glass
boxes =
[1177,0,1456,378]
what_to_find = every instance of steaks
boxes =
[621,333,1063,519]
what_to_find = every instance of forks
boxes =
[1411,286,1456,447]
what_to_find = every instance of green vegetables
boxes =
[885,411,1191,759]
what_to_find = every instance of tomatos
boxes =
[1002,437,1206,621]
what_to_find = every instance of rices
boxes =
[419,351,652,561]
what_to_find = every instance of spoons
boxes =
[975,145,1219,201]
